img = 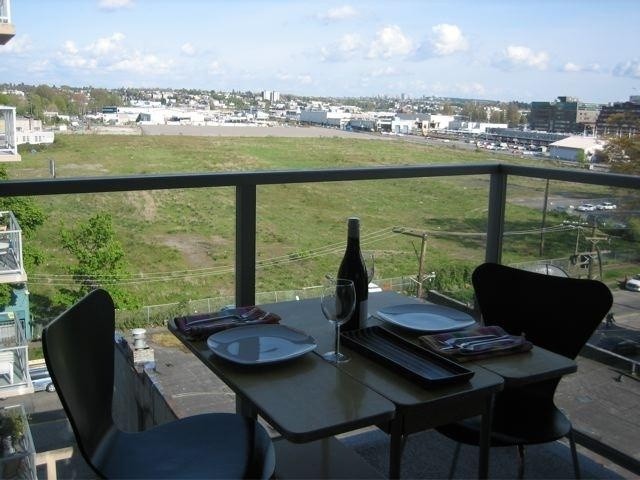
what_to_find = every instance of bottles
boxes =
[335,216,370,345]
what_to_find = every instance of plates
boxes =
[205,321,319,370]
[374,301,478,333]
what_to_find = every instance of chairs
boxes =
[416,263,614,479]
[42,290,275,480]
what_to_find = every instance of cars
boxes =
[577,203,596,212]
[468,139,538,156]
[597,202,617,210]
[425,135,450,143]
[625,272,640,293]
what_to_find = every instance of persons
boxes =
[606,313,616,328]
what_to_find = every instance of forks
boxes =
[182,306,279,329]
[437,332,528,356]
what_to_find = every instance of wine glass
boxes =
[319,277,358,366]
